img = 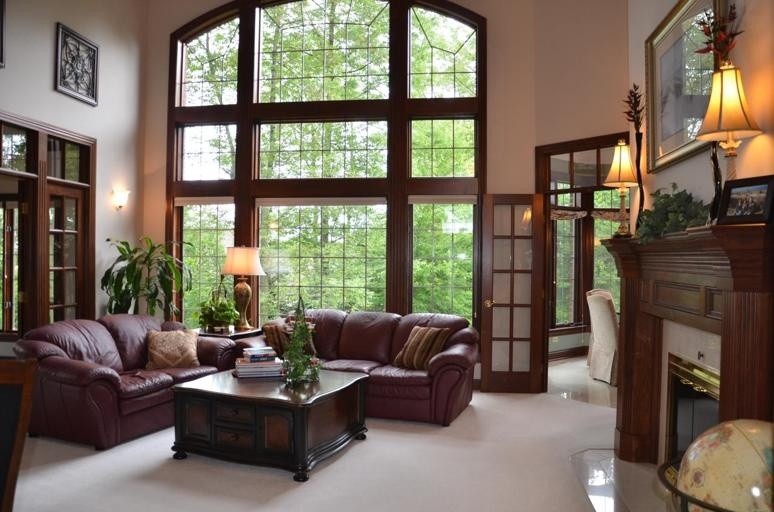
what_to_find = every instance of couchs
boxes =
[236,306,483,430]
[10,311,235,452]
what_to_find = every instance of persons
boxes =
[735,197,760,215]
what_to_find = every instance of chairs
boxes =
[586,288,616,385]
[1,348,41,512]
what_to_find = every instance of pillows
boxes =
[140,326,202,371]
[262,319,317,358]
[391,322,450,371]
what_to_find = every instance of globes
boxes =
[660,419,774,512]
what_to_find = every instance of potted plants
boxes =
[619,75,646,234]
[199,281,238,335]
[690,0,748,223]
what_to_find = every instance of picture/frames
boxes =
[49,20,101,108]
[642,0,729,174]
[713,173,773,226]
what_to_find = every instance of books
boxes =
[234,347,285,379]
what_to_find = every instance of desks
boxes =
[199,323,264,341]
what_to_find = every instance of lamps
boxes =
[598,139,637,239]
[694,57,765,180]
[221,241,266,330]
[110,182,129,209]
